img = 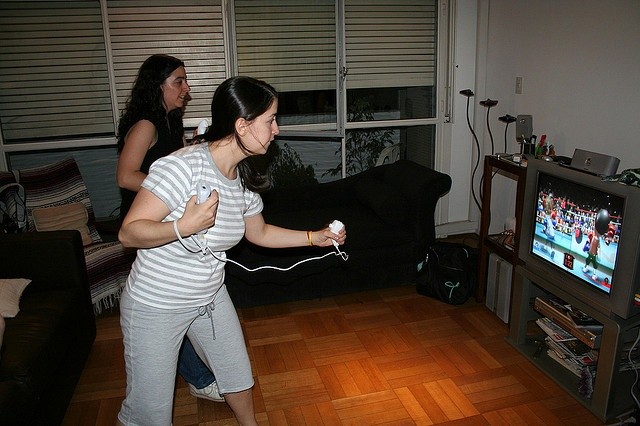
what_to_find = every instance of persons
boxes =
[116,75,347,426]
[537,190,619,283]
[116,53,224,402]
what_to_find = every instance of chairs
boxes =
[374,142,401,167]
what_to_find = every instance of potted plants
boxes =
[269,143,319,196]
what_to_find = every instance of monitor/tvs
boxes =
[518,156,640,320]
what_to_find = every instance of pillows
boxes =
[353,175,410,227]
[0,315,5,348]
[31,201,95,247]
[0,277,32,319]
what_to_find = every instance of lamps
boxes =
[461,85,517,215]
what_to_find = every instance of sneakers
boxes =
[189,380,225,402]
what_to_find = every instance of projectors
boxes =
[571,148,621,177]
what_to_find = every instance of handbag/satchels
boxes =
[414,259,475,304]
[428,241,484,285]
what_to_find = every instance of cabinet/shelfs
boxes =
[503,265,640,426]
[477,154,527,329]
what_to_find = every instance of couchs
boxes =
[0,229,98,426]
[224,159,453,309]
[0,155,134,316]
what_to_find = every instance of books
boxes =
[535,316,640,382]
